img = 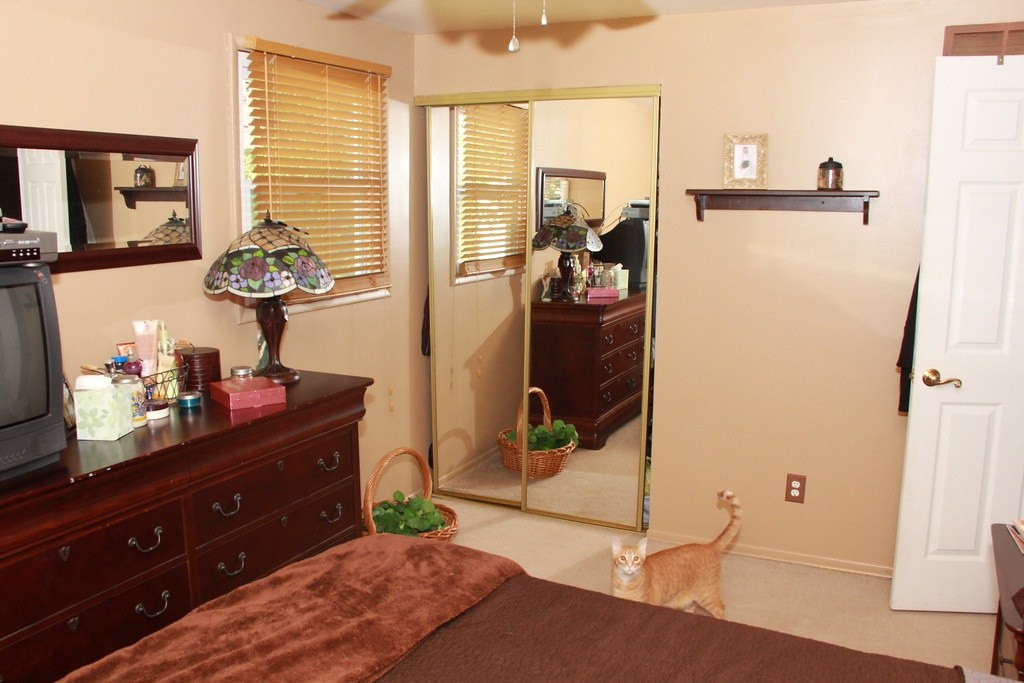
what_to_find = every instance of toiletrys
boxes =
[155,320,180,398]
[115,342,137,363]
[573,251,606,287]
[131,319,159,387]
[124,348,143,379]
[104,354,127,378]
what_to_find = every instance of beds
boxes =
[43,536,1024,683]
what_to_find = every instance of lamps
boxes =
[203,210,334,387]
[530,204,602,302]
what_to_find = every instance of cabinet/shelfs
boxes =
[529,286,645,449]
[0,364,374,683]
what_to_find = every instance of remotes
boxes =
[0,222,28,234]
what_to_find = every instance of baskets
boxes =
[499,386,576,479]
[361,447,457,543]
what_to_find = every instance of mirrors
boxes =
[0,125,203,274]
[417,97,661,529]
[536,167,606,227]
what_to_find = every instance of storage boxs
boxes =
[104,360,190,407]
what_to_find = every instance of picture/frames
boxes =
[722,133,770,189]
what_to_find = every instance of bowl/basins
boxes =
[3,223,28,232]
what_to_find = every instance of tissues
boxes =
[72,373,135,442]
[611,262,629,290]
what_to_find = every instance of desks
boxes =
[990,522,1024,681]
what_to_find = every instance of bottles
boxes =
[567,254,614,300]
[157,321,170,356]
[123,347,143,376]
[111,374,148,428]
[114,356,126,374]
[134,164,152,186]
[230,366,253,380]
[817,157,844,191]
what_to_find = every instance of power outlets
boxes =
[785,473,806,504]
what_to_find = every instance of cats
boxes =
[608,490,743,621]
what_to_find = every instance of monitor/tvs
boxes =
[593,217,650,288]
[0,262,67,481]
